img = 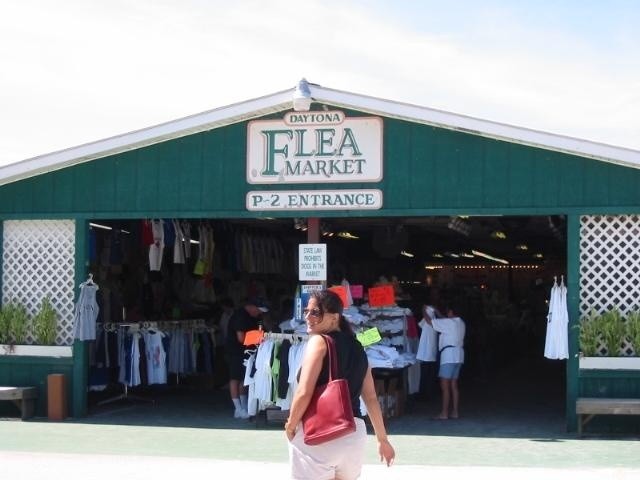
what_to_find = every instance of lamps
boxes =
[292,79,316,114]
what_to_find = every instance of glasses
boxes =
[304,308,331,318]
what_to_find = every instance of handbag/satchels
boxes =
[300,334,358,446]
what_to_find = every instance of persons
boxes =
[219,297,234,344]
[283,289,395,479]
[224,296,270,419]
[423,300,466,420]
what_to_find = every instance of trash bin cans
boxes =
[49,374,66,419]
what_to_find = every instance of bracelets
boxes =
[285,422,295,434]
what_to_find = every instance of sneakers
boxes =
[234,404,251,419]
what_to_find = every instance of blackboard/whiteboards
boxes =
[298,244,327,281]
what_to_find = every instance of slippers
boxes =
[430,410,459,420]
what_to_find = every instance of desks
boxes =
[371,366,413,419]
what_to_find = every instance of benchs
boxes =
[0,386,40,421]
[575,397,640,439]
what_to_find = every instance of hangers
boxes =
[126,320,217,339]
[244,331,309,357]
[145,218,216,233]
[549,274,567,292]
[79,274,100,290]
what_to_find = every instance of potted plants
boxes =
[574,304,639,370]
[1,294,73,358]
